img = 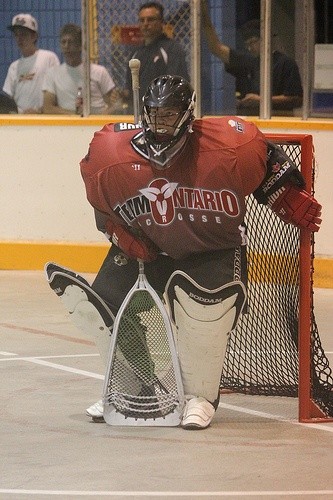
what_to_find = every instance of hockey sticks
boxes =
[103,59,185,427]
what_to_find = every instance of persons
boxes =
[80,74,321,430]
[109,3,190,114]
[0,12,59,114]
[41,23,124,116]
[201,2,304,117]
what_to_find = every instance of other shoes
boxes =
[86,398,105,423]
[180,397,215,430]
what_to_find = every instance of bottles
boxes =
[76,88,83,115]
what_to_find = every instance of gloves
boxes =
[103,219,158,262]
[267,182,322,234]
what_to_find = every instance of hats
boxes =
[5,12,38,36]
[240,19,277,40]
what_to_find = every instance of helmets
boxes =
[141,75,196,156]
